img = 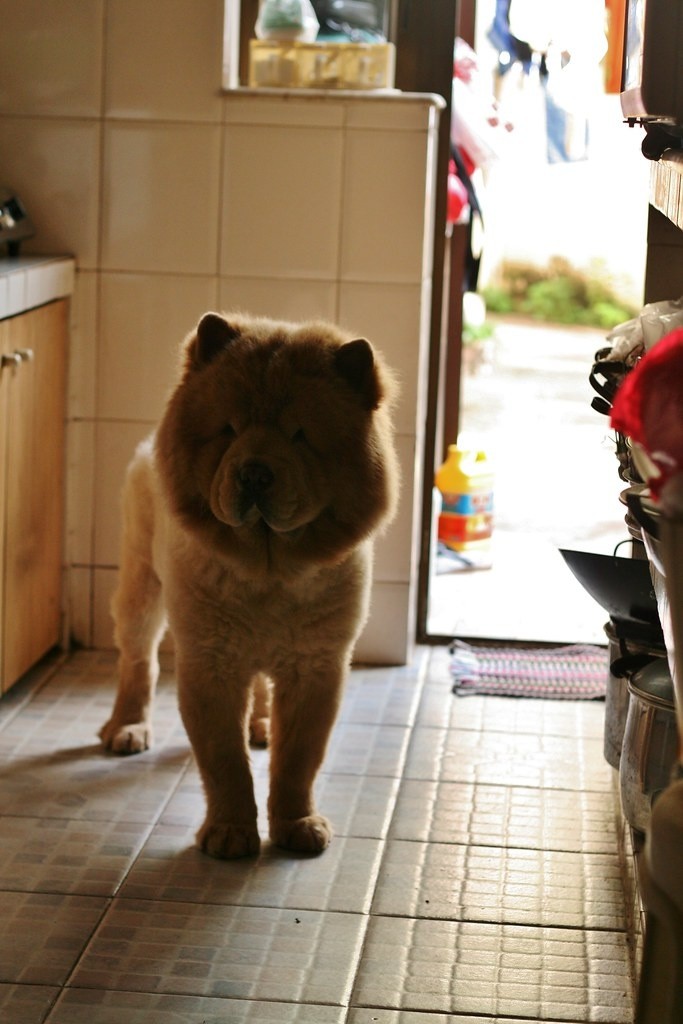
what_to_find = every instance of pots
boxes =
[611,658,683,832]
[558,548,661,627]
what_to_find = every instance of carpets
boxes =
[450,640,608,700]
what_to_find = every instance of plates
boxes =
[618,468,661,544]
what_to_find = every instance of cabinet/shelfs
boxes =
[1,297,71,700]
[618,0,683,1024]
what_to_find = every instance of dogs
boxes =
[95,312,404,862]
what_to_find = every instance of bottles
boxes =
[436,445,495,550]
